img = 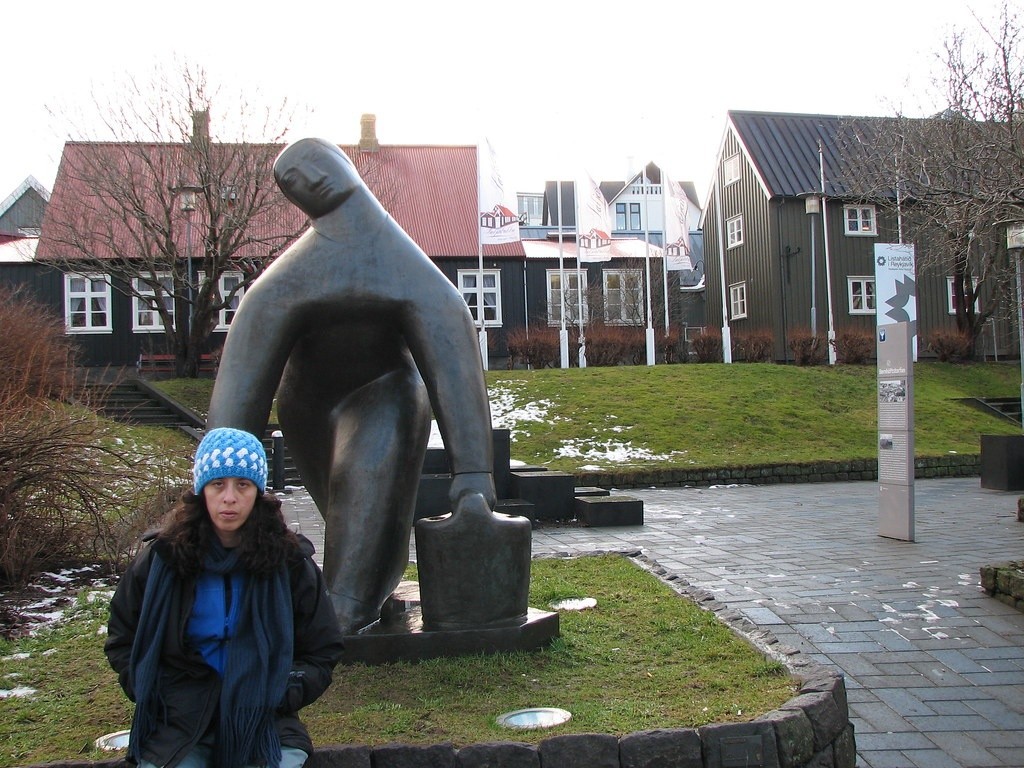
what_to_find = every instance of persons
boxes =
[208,135,498,634]
[104,428,345,768]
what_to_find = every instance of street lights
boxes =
[168,183,210,375]
[798,188,831,366]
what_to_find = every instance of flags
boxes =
[575,168,612,262]
[477,144,520,244]
[661,171,692,271]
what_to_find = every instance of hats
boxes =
[194,428,267,495]
